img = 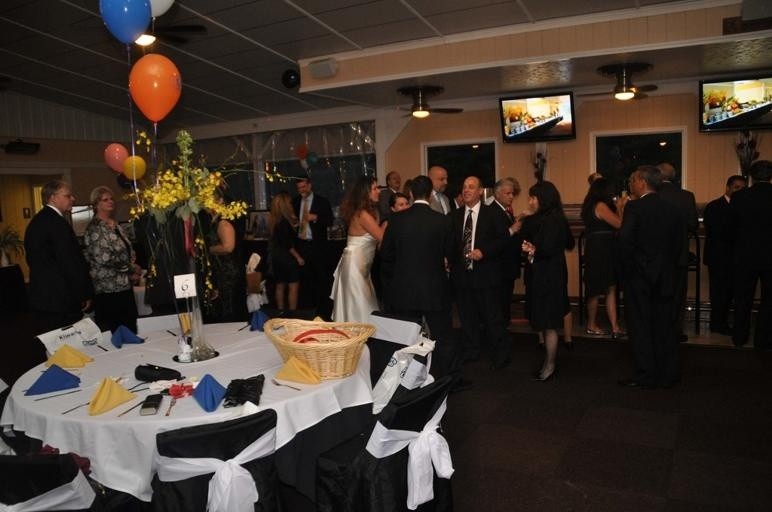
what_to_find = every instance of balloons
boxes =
[296,143,319,171]
[103,142,147,189]
[281,69,300,89]
[149,0,176,17]
[129,53,182,123]
[98,0,151,46]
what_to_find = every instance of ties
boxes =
[436,194,446,216]
[463,209,473,270]
[301,200,309,239]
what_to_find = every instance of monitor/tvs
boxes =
[698,75,772,133]
[499,91,576,144]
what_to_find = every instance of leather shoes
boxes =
[527,360,558,384]
[562,338,573,348]
[613,330,628,339]
[583,327,609,335]
[446,372,477,393]
[617,374,672,390]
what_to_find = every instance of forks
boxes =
[271,378,301,391]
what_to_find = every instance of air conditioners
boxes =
[80,16,212,49]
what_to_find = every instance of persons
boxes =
[264,191,310,319]
[701,174,749,339]
[191,183,250,324]
[581,163,701,388]
[327,164,575,381]
[80,186,142,337]
[21,178,93,330]
[724,158,772,353]
[130,192,192,314]
[290,174,336,311]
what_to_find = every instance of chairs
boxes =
[505,229,704,336]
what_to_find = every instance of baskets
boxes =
[264,317,377,378]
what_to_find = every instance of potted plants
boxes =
[0,221,27,288]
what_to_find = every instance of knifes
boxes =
[166,398,176,417]
[117,400,144,417]
[33,389,80,401]
[61,402,89,415]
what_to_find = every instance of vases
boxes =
[181,246,219,362]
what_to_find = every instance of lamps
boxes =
[611,84,637,99]
[410,104,432,118]
[134,28,156,48]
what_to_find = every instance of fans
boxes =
[394,86,464,118]
[574,66,659,101]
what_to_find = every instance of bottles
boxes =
[178,336,193,361]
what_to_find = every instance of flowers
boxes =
[120,123,303,307]
[527,147,549,183]
[731,129,762,174]
